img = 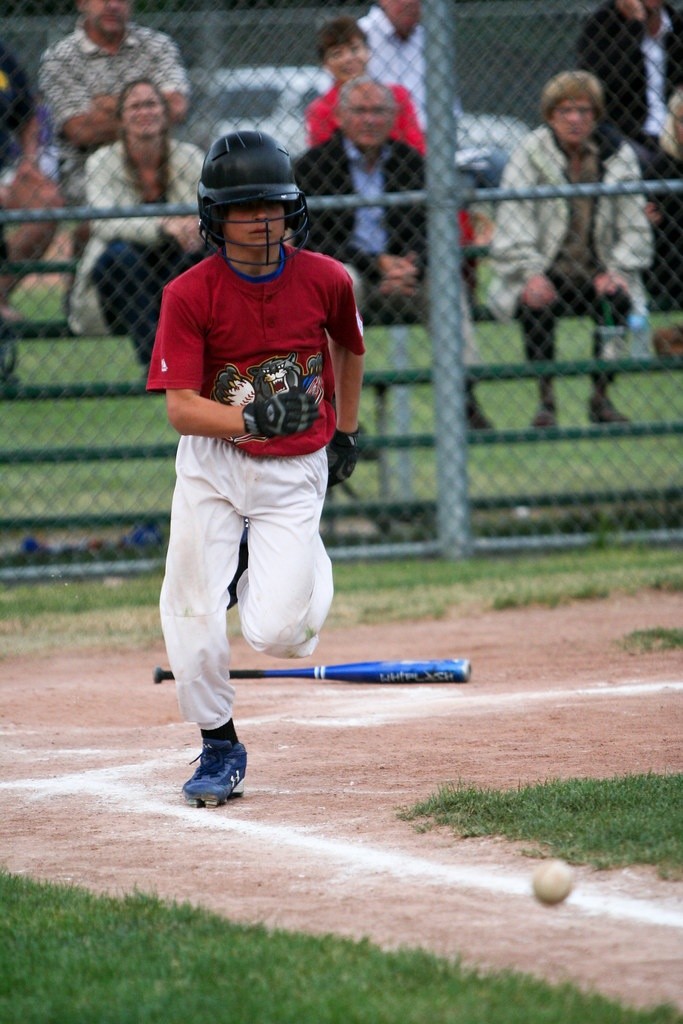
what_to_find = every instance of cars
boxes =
[166,65,534,171]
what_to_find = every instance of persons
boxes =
[67,81,213,371]
[488,71,654,429]
[305,0,475,240]
[38,0,192,314]
[145,132,365,810]
[577,0,683,307]
[295,79,492,431]
[1,41,64,318]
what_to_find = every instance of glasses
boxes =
[554,107,595,117]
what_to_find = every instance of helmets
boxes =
[197,131,309,232]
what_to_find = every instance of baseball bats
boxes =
[152,658,472,685]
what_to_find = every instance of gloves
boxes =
[243,393,319,438]
[327,423,362,487]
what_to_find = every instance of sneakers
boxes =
[183,739,247,808]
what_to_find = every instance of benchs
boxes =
[0,250,682,524]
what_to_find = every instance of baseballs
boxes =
[533,858,576,904]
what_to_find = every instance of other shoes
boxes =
[469,411,490,428]
[532,403,557,426]
[590,394,628,422]
